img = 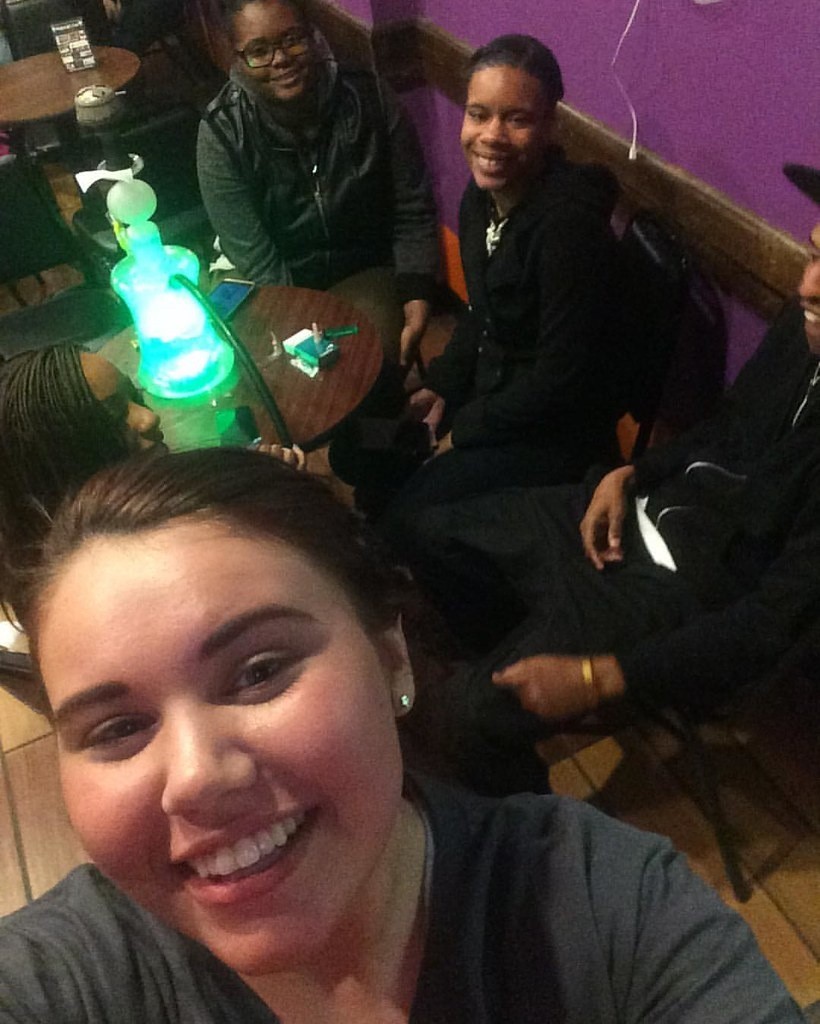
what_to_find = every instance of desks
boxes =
[0,45,142,284]
[93,282,384,455]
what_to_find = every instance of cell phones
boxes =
[205,278,256,324]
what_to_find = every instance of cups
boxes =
[75,85,128,214]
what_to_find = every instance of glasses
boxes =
[232,28,312,68]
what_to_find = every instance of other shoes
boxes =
[423,599,527,658]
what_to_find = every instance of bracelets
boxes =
[581,655,600,710]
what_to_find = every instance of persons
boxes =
[0,0,820,1024]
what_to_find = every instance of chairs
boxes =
[0,0,764,904]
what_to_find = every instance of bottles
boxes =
[109,221,234,399]
